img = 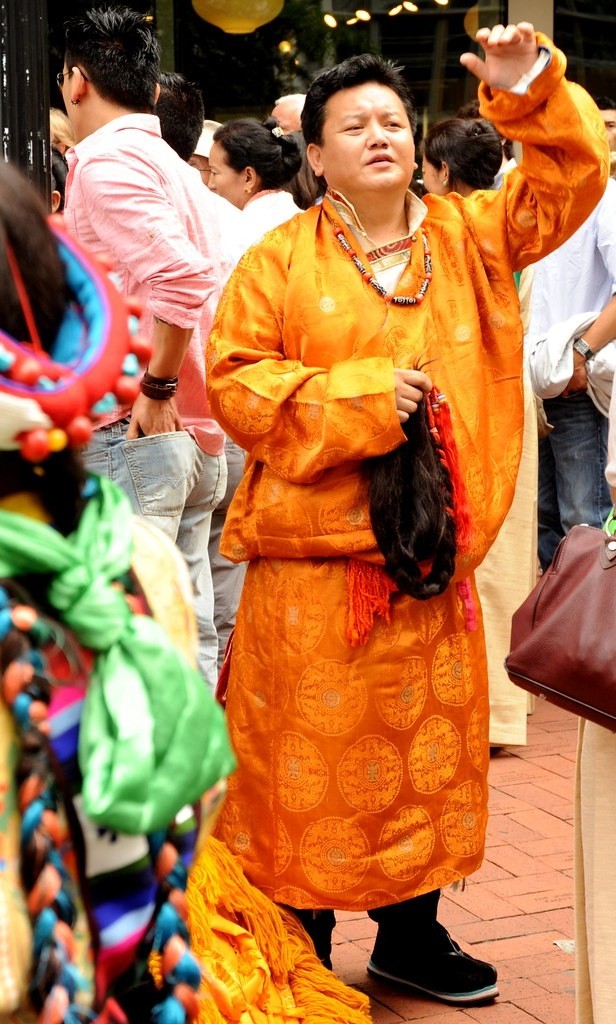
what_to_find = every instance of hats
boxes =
[193,120,222,157]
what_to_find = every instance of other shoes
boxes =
[298,914,335,972]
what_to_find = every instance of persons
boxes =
[158,84,183,161]
[412,118,531,759]
[49,144,69,217]
[203,113,339,703]
[154,69,254,339]
[409,176,425,200]
[0,149,220,1024]
[188,118,229,203]
[49,104,79,165]
[51,5,226,751]
[508,118,616,578]
[282,130,322,209]
[591,100,616,183]
[268,92,327,207]
[507,141,522,174]
[183,10,614,1011]
[457,99,511,205]
[605,349,615,533]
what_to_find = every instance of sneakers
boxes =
[366,925,497,1000]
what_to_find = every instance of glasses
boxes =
[55,65,88,92]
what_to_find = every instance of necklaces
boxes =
[318,189,436,305]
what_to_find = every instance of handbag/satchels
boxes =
[505,509,615,731]
